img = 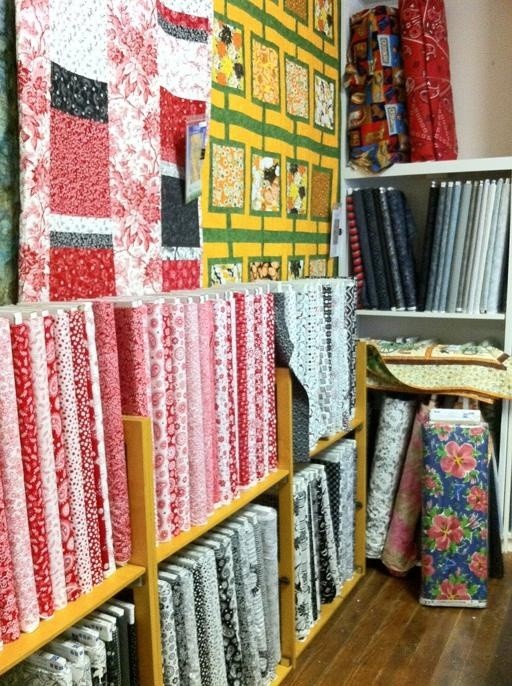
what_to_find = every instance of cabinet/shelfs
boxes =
[345,155,512,321]
[2,342,370,685]
